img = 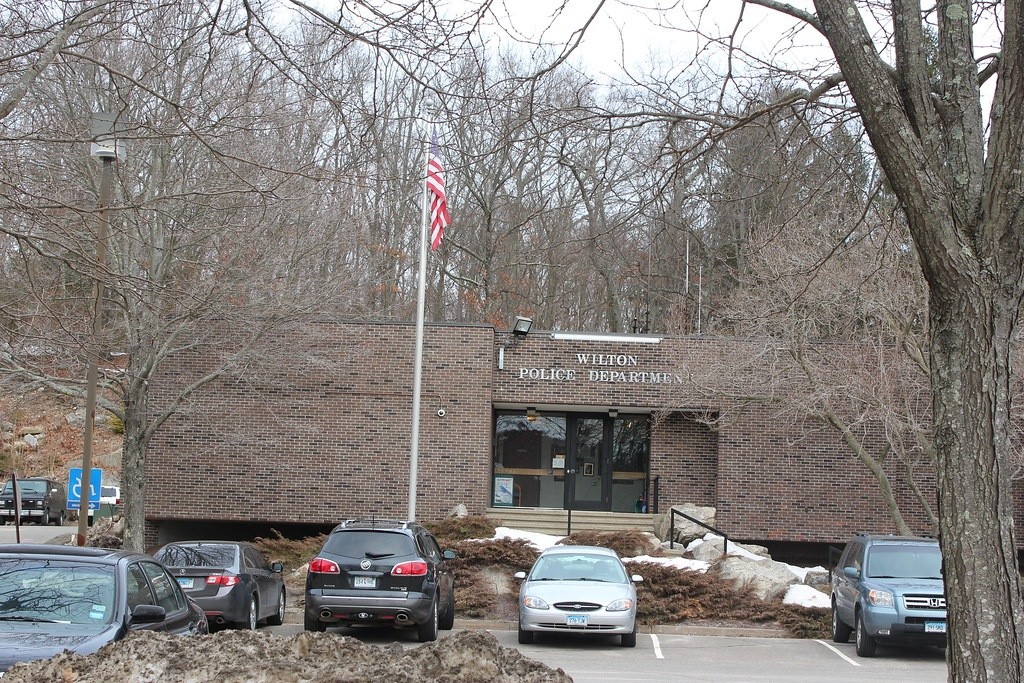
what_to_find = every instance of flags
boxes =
[426,123,452,250]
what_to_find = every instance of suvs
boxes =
[831,531,948,657]
[304,519,455,643]
[0,478,67,526]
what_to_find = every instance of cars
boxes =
[78,485,121,526]
[153,540,287,631]
[0,544,210,674]
[514,545,644,648]
